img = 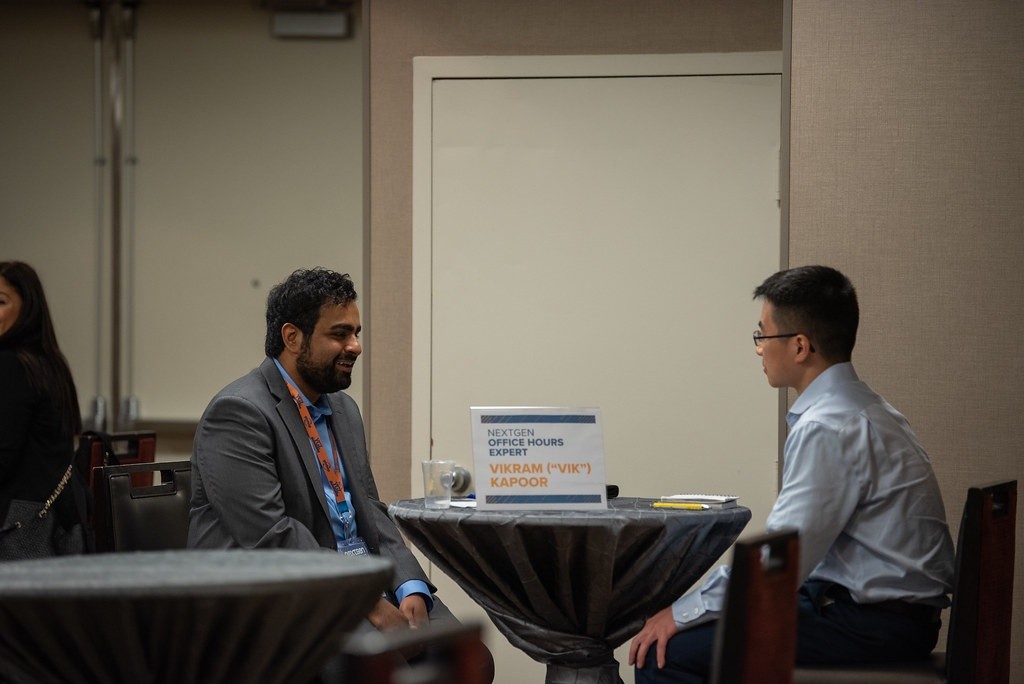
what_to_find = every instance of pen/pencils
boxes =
[650,501,711,510]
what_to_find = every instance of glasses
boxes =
[753,330,816,352]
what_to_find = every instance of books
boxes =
[659,493,739,509]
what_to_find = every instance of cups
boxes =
[422,460,454,509]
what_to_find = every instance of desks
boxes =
[387,496,752,684]
[0,545,398,684]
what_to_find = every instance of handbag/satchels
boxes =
[0,430,114,559]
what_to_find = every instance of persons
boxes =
[0,262,87,558]
[184,265,494,684]
[628,265,952,684]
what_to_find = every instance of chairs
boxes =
[792,478,1018,684]
[93,460,193,553]
[711,526,801,684]
[78,430,157,528]
